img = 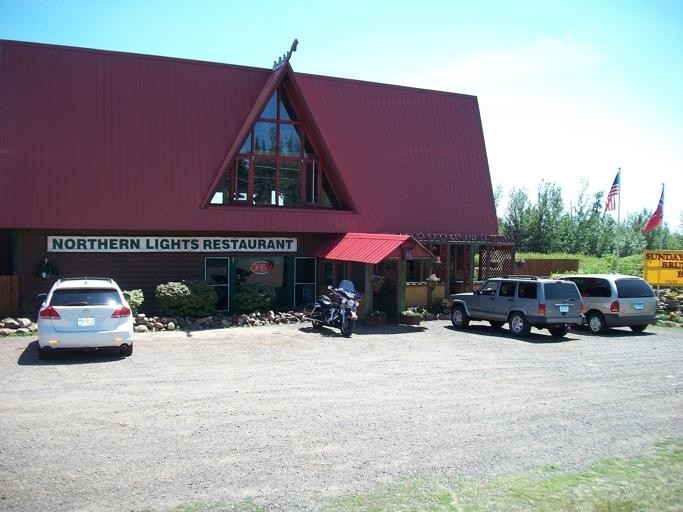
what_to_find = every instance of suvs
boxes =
[448,275,589,339]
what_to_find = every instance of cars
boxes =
[36,277,133,359]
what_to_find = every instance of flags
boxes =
[603,170,619,212]
[642,184,663,235]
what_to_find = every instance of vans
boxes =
[557,274,660,335]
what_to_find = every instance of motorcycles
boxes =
[302,280,362,337]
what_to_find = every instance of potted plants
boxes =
[425,273,441,290]
[400,310,422,325]
